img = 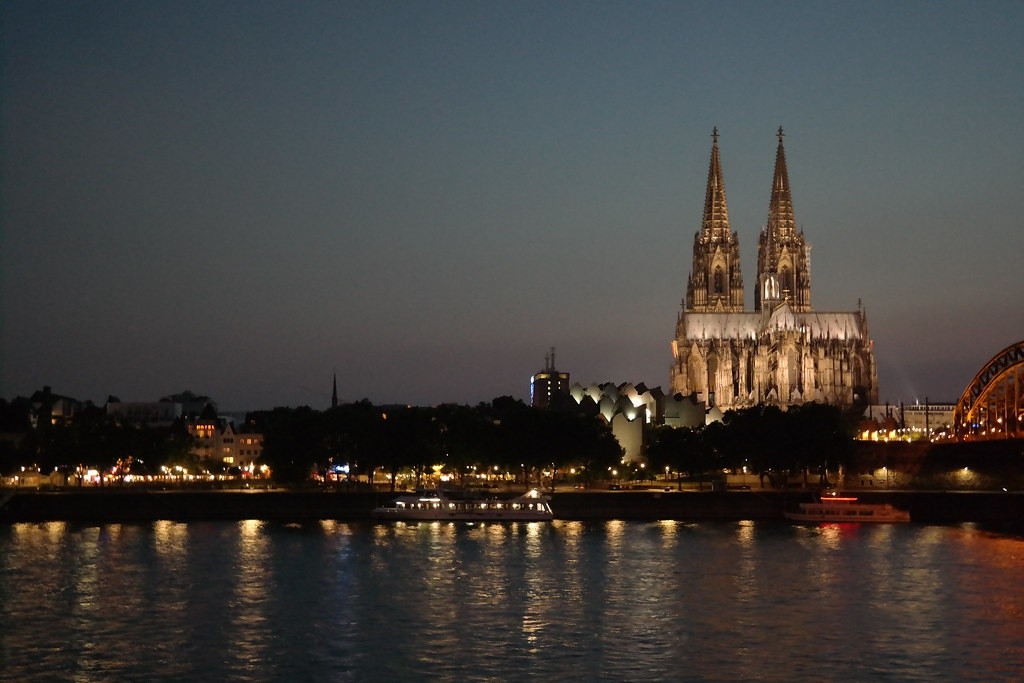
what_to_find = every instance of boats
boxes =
[370,484,557,521]
[778,495,911,523]
